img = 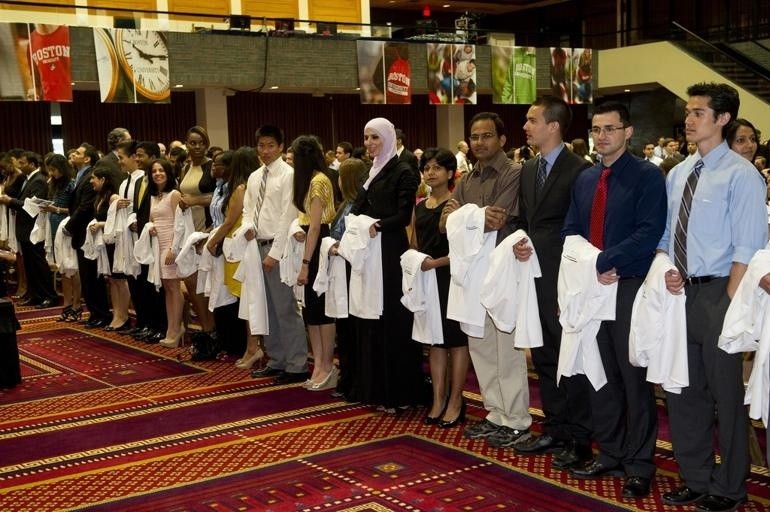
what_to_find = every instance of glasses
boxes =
[589,125,630,134]
[469,133,499,140]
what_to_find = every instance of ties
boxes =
[535,156,547,197]
[124,174,131,197]
[673,161,705,281]
[137,177,146,208]
[252,167,270,235]
[590,170,612,250]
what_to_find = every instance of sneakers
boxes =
[464,418,532,448]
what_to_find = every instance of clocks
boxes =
[116,28,170,101]
[94,27,119,103]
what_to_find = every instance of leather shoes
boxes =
[514,434,569,454]
[253,366,305,384]
[16,299,58,309]
[697,494,749,510]
[118,325,164,343]
[77,311,112,330]
[661,486,706,503]
[618,478,653,497]
[552,443,622,477]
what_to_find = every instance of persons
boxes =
[370,41,411,104]
[550,47,569,104]
[23,24,73,102]
[0,80,769,512]
[426,43,477,104]
[572,47,593,104]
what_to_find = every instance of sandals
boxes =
[376,400,412,416]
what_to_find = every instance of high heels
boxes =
[160,324,186,347]
[56,305,84,323]
[422,395,466,428]
[299,363,340,391]
[233,347,264,370]
[102,317,131,330]
